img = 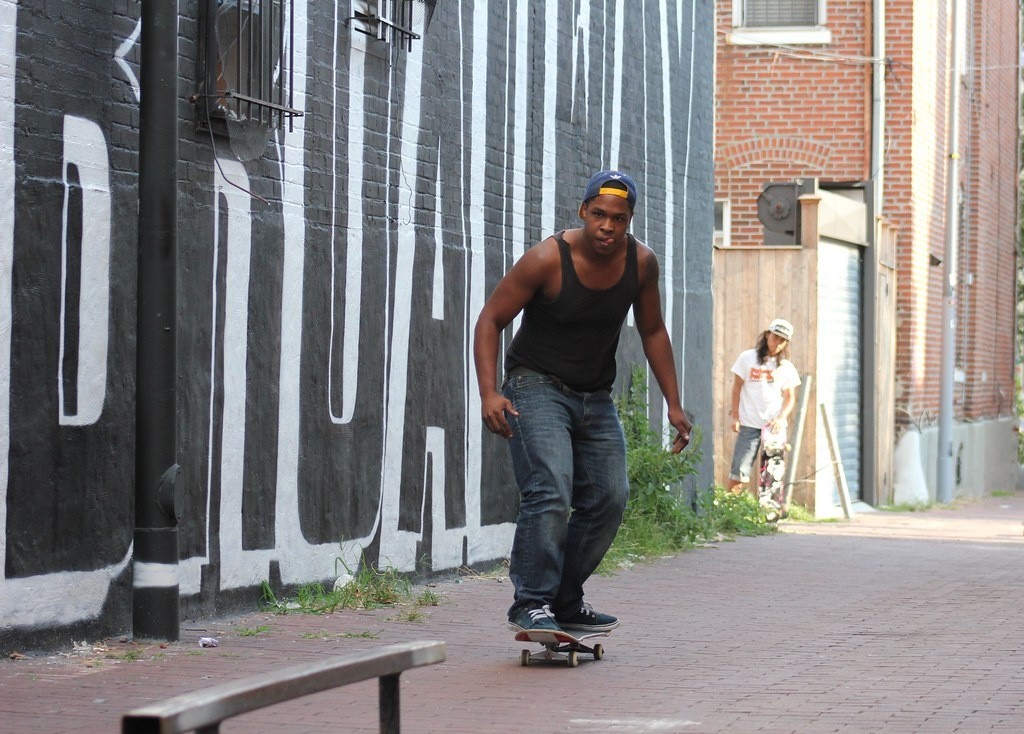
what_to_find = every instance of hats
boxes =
[578,170,637,221]
[768,318,794,342]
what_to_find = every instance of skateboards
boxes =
[515,628,612,667]
[760,423,792,524]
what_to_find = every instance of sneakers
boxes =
[506,601,563,634]
[553,604,622,632]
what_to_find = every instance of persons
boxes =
[474,170,694,632]
[727,317,802,492]
